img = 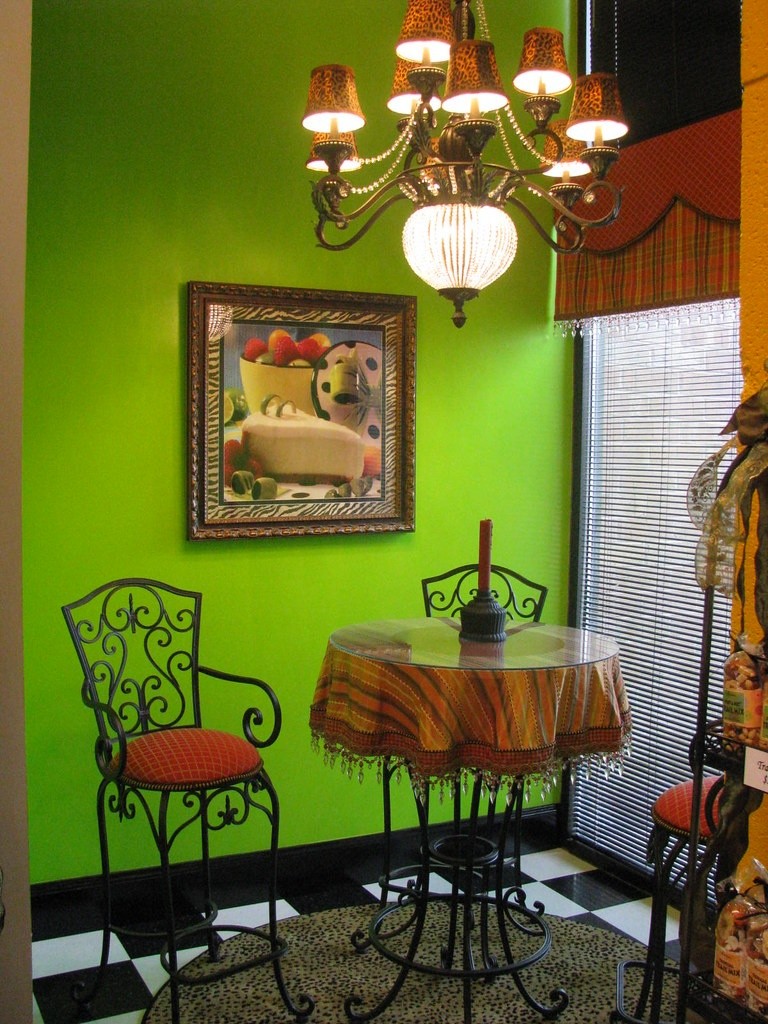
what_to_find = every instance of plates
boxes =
[310,340,381,480]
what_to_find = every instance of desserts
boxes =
[241,393,364,485]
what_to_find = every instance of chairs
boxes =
[62,578,315,1024]
[378,564,548,926]
[609,716,724,1024]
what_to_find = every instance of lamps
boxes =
[301,0,629,329]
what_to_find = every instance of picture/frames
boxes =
[187,280,418,541]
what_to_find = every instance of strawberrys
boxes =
[221,438,263,489]
[244,335,323,369]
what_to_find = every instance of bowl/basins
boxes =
[239,352,314,417]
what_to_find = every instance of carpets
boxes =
[142,902,706,1024]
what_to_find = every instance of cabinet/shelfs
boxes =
[675,445,768,1024]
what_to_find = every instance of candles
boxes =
[478,517,492,592]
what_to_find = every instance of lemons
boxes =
[224,386,249,426]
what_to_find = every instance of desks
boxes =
[310,616,633,1024]
